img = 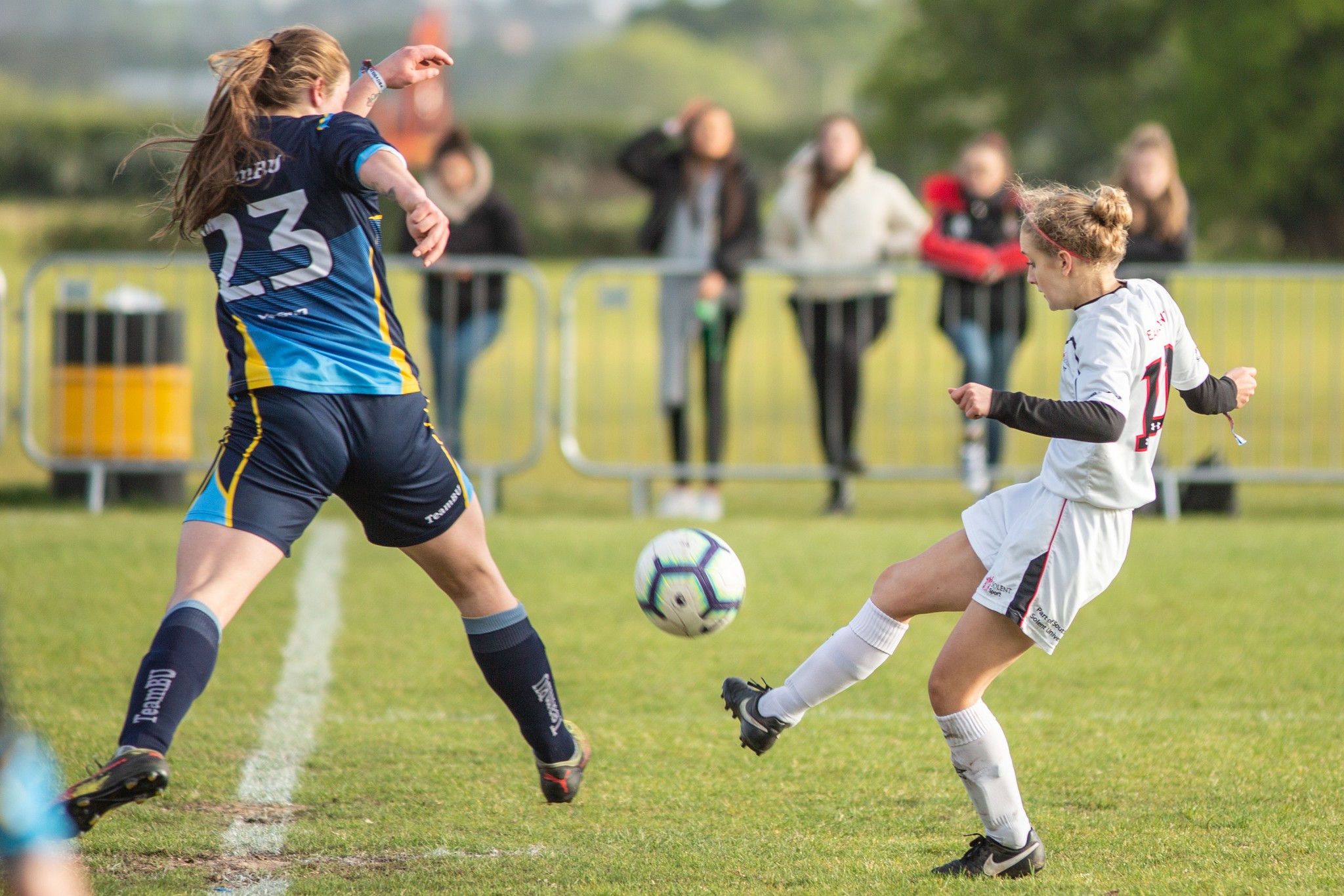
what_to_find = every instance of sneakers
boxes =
[930,826,1046,879]
[535,719,591,805]
[720,676,792,757]
[51,747,169,840]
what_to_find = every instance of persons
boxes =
[918,130,1033,498]
[396,125,531,479]
[612,97,767,521]
[58,23,589,836]
[720,175,1260,880]
[1106,120,1195,286]
[767,111,933,523]
[0,679,90,896]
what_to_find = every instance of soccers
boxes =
[632,527,746,638]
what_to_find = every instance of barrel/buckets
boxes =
[50,304,190,504]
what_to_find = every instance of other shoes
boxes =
[657,485,724,522]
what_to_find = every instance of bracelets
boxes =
[357,58,386,94]
